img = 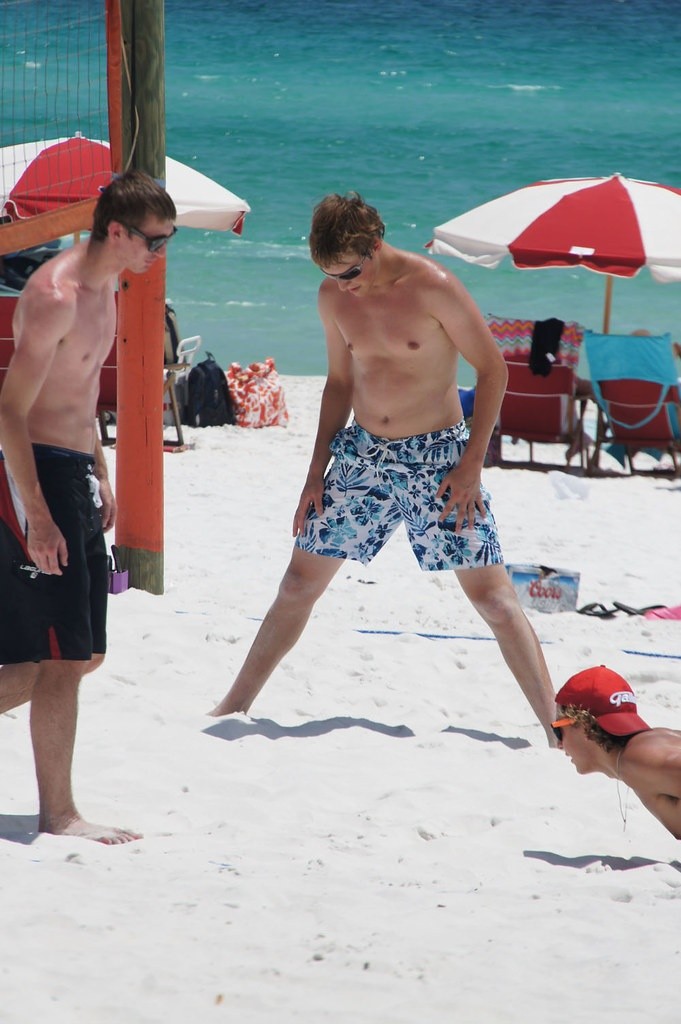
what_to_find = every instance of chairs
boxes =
[582,329,681,479]
[0,297,191,454]
[484,313,595,472]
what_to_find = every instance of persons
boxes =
[499,318,570,444]
[552,666,681,842]
[208,193,558,749]
[0,171,179,845]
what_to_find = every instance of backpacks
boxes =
[185,351,237,428]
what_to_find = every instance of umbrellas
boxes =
[0,132,252,255]
[423,173,680,335]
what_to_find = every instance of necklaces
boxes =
[616,750,629,832]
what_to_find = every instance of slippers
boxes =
[577,603,618,616]
[614,601,666,615]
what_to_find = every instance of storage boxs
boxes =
[505,563,580,614]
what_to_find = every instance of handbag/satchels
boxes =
[225,357,288,428]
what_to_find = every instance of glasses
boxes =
[320,249,369,280]
[128,225,178,252]
[549,717,579,740]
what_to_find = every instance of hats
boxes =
[555,664,653,736]
